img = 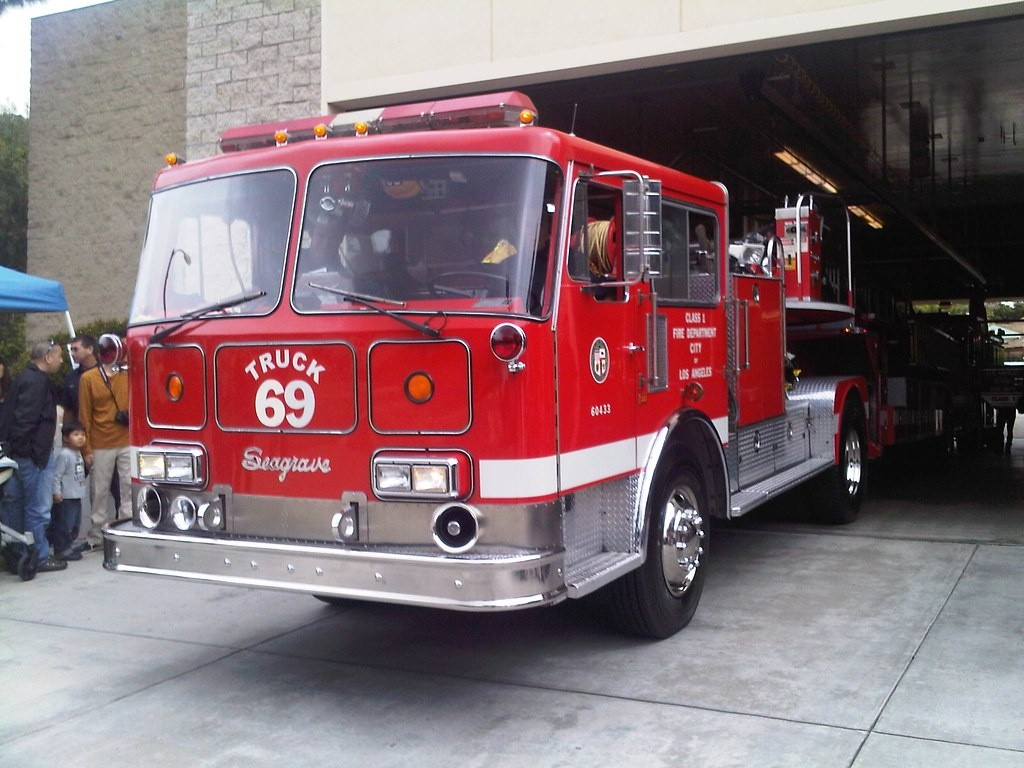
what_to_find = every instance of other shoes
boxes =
[57,552,83,561]
[73,542,102,553]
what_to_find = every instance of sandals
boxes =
[36,555,68,572]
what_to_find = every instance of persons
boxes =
[487,201,596,321]
[997,408,1024,458]
[0,332,138,579]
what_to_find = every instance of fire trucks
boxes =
[97,90,897,641]
[827,254,1001,449]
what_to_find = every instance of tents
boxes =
[0,265,77,338]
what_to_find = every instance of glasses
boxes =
[47,338,56,354]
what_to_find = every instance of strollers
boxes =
[0,441,43,582]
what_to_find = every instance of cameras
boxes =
[116,411,130,427]
